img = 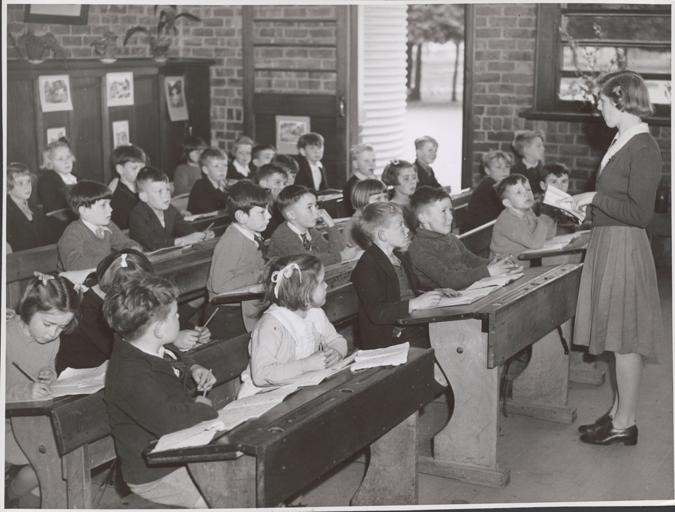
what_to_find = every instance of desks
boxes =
[6,184,592,508]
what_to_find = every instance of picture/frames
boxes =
[24,4,89,25]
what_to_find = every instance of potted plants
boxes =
[18,31,62,65]
[124,5,202,63]
[89,31,122,63]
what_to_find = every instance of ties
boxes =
[255,235,266,257]
[300,232,310,250]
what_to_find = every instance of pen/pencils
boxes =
[500,255,514,263]
[11,361,53,396]
[319,342,323,351]
[417,288,426,293]
[204,222,215,233]
[202,368,212,399]
[200,307,219,333]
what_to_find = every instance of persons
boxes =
[538,164,577,227]
[350,201,434,351]
[61,248,211,367]
[101,273,217,506]
[207,180,345,301]
[236,253,348,397]
[559,70,663,445]
[469,131,548,226]
[6,269,89,509]
[490,174,558,269]
[407,184,519,291]
[341,135,451,249]
[5,132,329,272]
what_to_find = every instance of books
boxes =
[151,341,410,453]
[51,359,109,397]
[524,230,591,251]
[437,265,525,308]
[541,184,587,224]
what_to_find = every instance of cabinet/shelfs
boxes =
[518,3,670,127]
[8,56,214,187]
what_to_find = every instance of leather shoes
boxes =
[580,421,638,445]
[579,415,612,432]
